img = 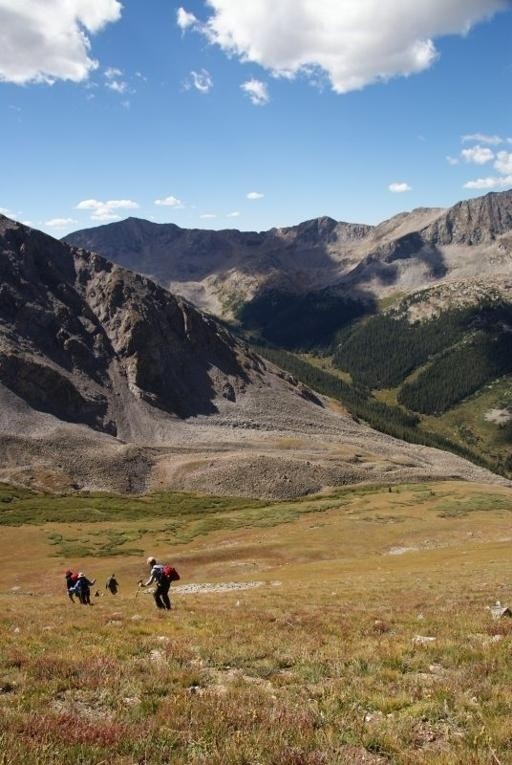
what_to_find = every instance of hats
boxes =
[78,573,85,578]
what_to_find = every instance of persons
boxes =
[105,573,119,596]
[65,569,96,605]
[139,557,172,609]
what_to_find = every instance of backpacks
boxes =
[161,566,180,580]
